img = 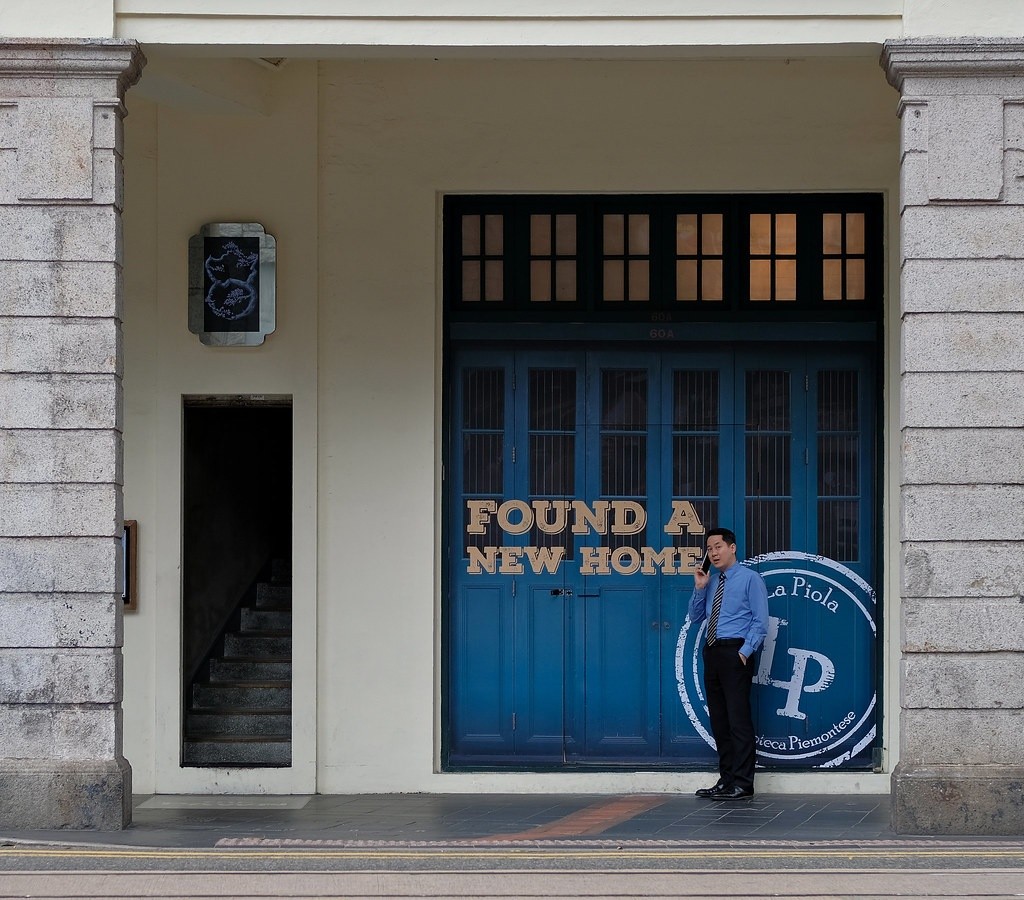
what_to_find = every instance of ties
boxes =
[707,572,727,646]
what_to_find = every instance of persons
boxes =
[689,528,768,800]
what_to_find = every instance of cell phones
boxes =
[700,551,711,576]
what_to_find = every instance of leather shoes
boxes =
[695,778,755,801]
